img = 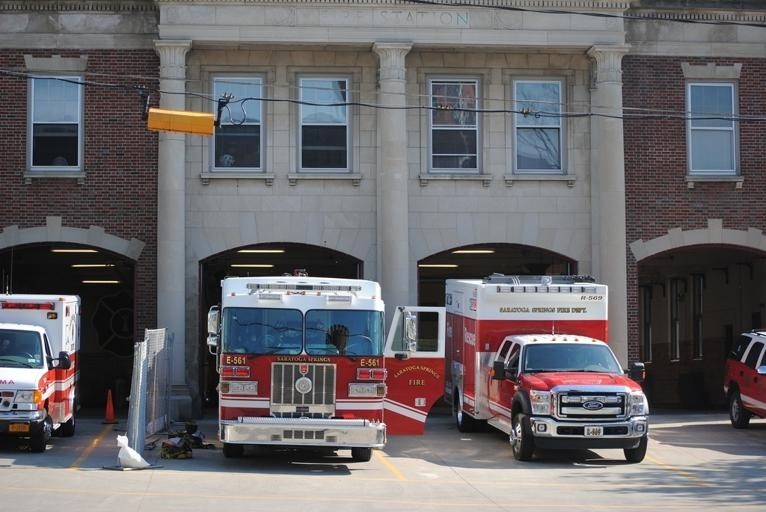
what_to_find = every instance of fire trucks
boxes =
[0,275,82,453]
[207,268,447,461]
[443,273,649,463]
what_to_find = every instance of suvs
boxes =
[722,329,766,429]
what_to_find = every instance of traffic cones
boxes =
[101,389,119,424]
